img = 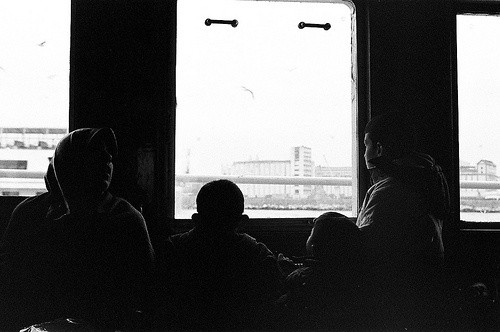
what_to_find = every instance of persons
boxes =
[266,209,370,312]
[155,179,282,332]
[350,109,457,295]
[0,128,160,332]
